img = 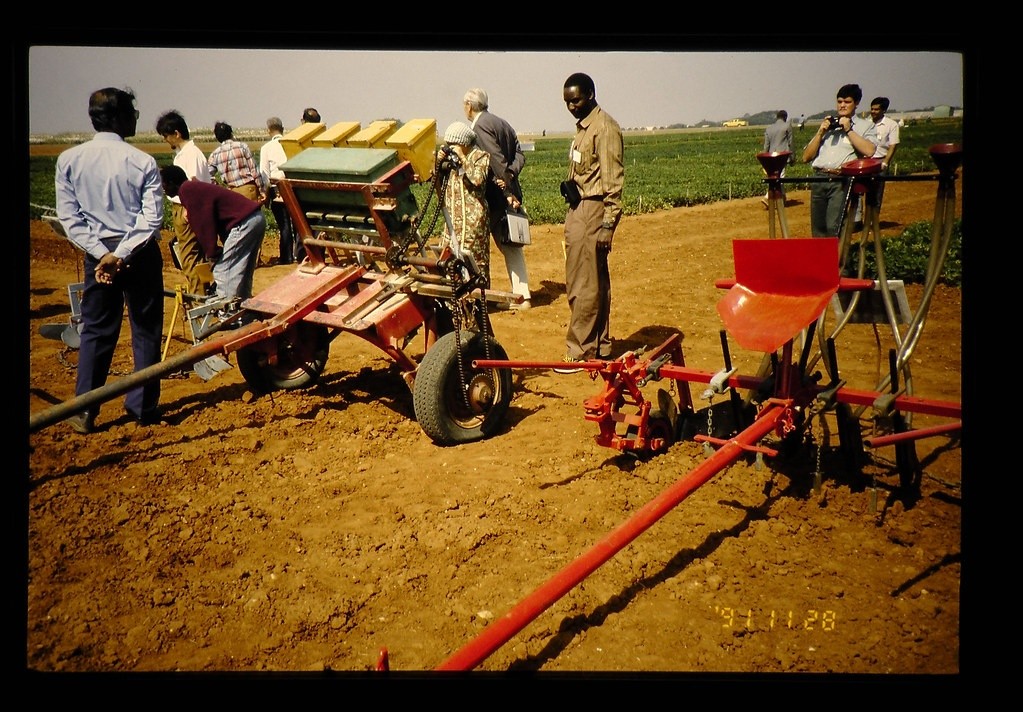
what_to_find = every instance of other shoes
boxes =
[852,221,863,232]
[761,197,769,206]
[64,413,94,433]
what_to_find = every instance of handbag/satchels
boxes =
[500,203,531,246]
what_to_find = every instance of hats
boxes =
[444,122,477,147]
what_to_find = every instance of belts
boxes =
[821,168,841,174]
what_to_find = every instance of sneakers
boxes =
[496,299,531,309]
[553,358,585,373]
[599,355,612,363]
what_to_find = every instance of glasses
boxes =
[128,110,139,119]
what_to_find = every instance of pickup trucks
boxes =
[722,119,749,128]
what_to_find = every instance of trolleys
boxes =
[373,141,959,673]
[29,121,524,447]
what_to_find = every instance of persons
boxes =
[860,97,900,224]
[434,123,494,334]
[799,113,806,133]
[552,73,625,372]
[462,90,532,312]
[803,84,878,238]
[300,108,321,127]
[55,87,163,435]
[207,122,266,209]
[259,117,310,265]
[761,110,793,210]
[156,109,218,309]
[160,166,266,329]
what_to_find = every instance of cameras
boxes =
[827,116,843,130]
[442,146,454,154]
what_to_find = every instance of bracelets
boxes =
[883,163,889,167]
[846,126,852,133]
